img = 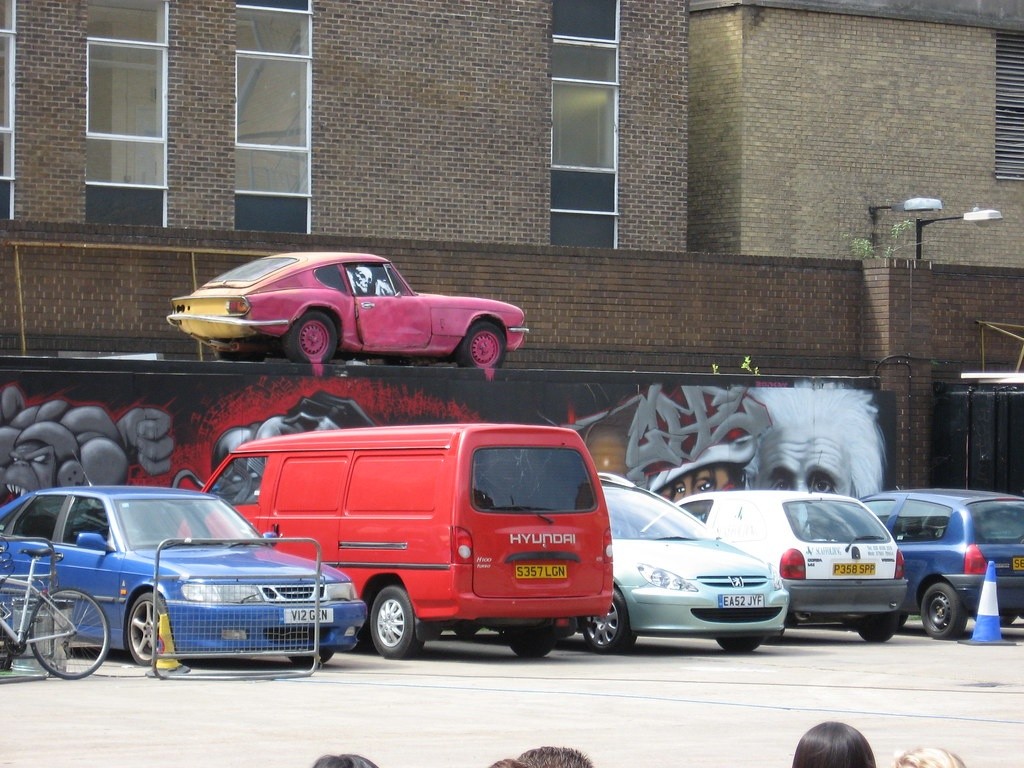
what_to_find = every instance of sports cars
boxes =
[165,248,532,371]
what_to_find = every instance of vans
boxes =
[175,423,617,662]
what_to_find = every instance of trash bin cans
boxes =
[11,595,74,674]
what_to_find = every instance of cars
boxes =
[0,483,369,669]
[634,489,908,642]
[858,487,1024,642]
[564,470,794,657]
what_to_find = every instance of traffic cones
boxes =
[954,557,1019,647]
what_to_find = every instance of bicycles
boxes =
[0,537,111,681]
